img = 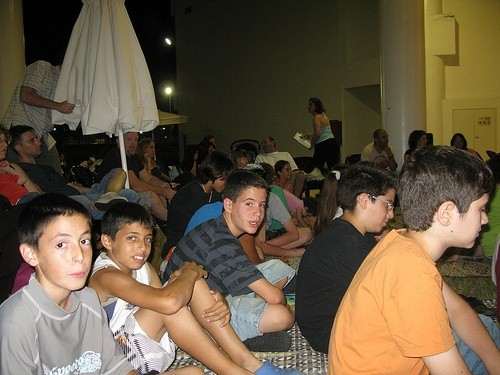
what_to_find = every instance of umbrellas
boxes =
[52,0,159,188]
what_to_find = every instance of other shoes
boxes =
[94,192,128,212]
[255,358,304,375]
[243,331,294,352]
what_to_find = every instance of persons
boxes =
[291,166,396,352]
[299,98,338,172]
[0,124,500,375]
[162,169,297,352]
[359,129,396,170]
[4,61,76,176]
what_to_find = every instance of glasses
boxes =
[367,195,394,212]
